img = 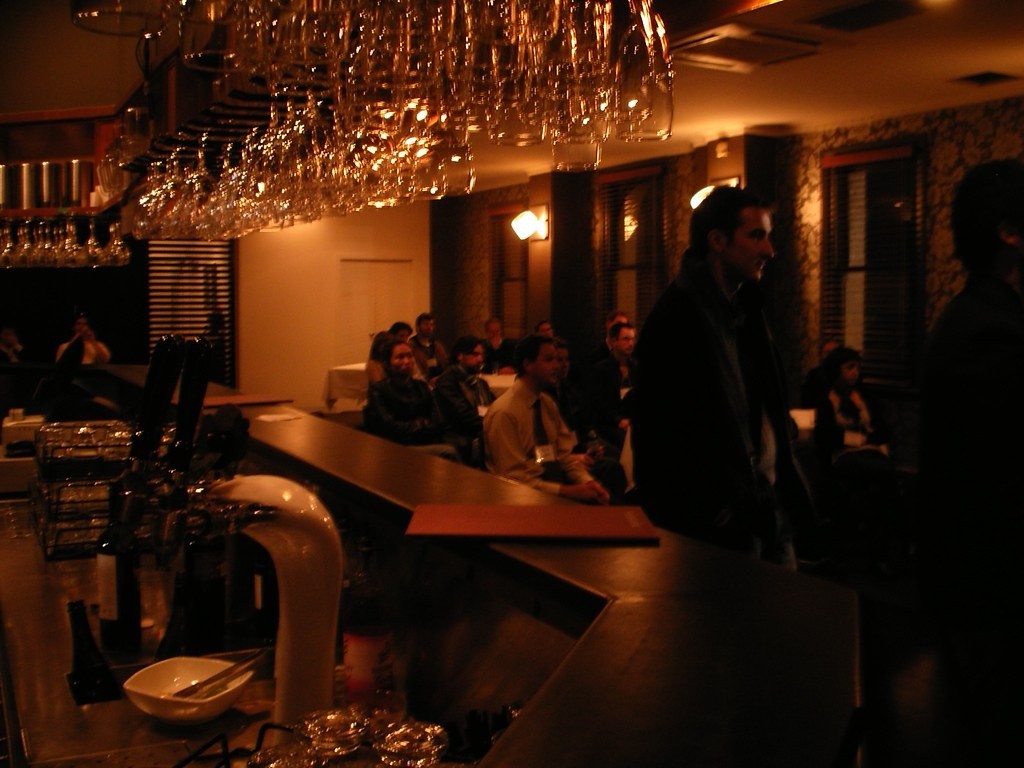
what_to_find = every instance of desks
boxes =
[322,362,369,410]
[789,408,816,442]
[474,373,516,399]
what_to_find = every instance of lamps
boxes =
[511,203,549,242]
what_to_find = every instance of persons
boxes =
[361,157,1024,768]
[0,312,120,410]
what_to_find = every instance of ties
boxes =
[471,377,488,407]
[534,398,561,482]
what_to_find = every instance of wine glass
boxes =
[129,0,673,240]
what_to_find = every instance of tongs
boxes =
[173,647,274,700]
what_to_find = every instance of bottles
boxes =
[96,490,140,664]
[0,218,130,267]
[67,600,125,703]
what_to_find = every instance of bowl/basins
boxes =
[123,656,254,724]
